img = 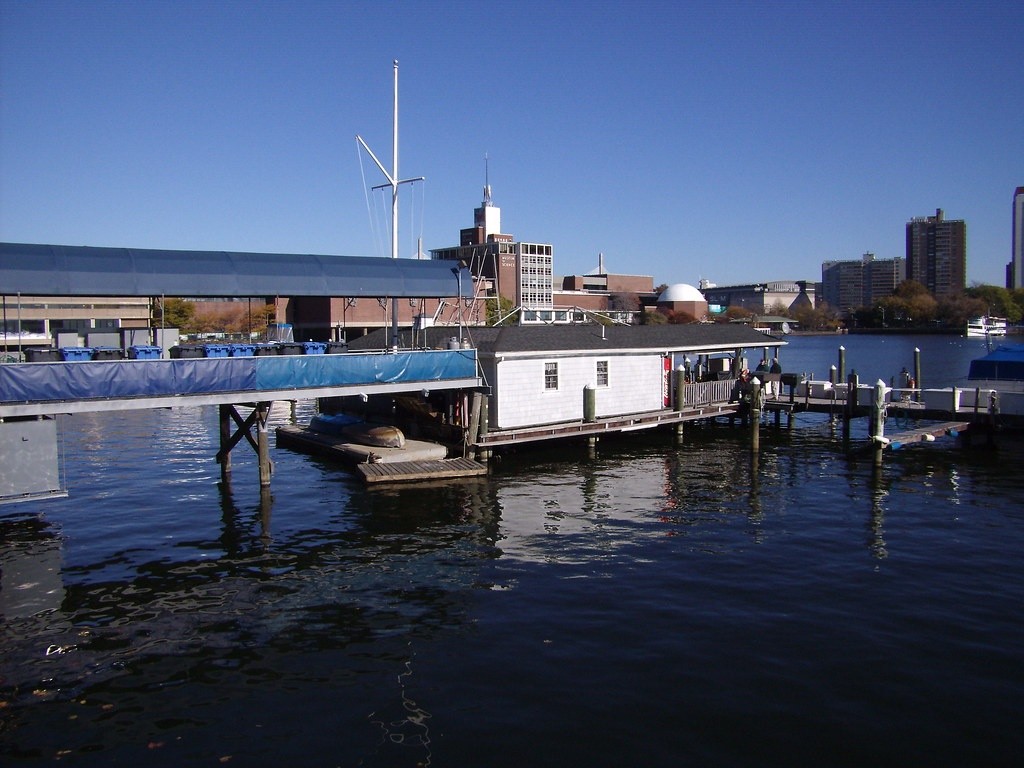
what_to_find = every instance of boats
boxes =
[310,412,406,448]
[966,315,1007,337]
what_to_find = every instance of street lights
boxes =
[879,306,885,327]
[848,307,854,327]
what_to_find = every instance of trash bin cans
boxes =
[323,341,350,354]
[126,345,163,359]
[89,345,124,360]
[274,342,304,355]
[256,342,280,356]
[300,342,327,355]
[199,343,231,357]
[168,345,204,359]
[22,347,60,362]
[57,346,94,362]
[224,343,258,357]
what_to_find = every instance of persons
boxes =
[756,359,770,383]
[769,358,781,400]
